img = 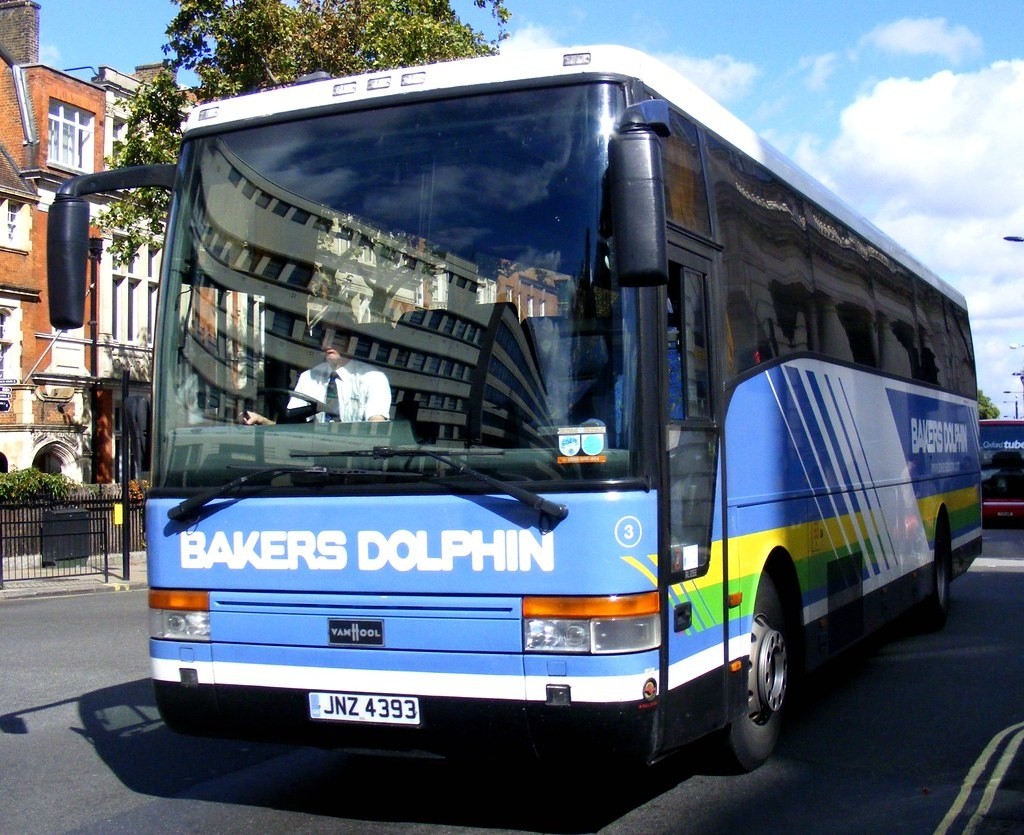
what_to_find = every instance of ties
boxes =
[323,371,341,422]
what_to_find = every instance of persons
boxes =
[238,313,392,424]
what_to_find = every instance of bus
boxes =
[975,418,1023,523]
[46,37,986,774]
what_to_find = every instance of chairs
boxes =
[521,316,700,447]
